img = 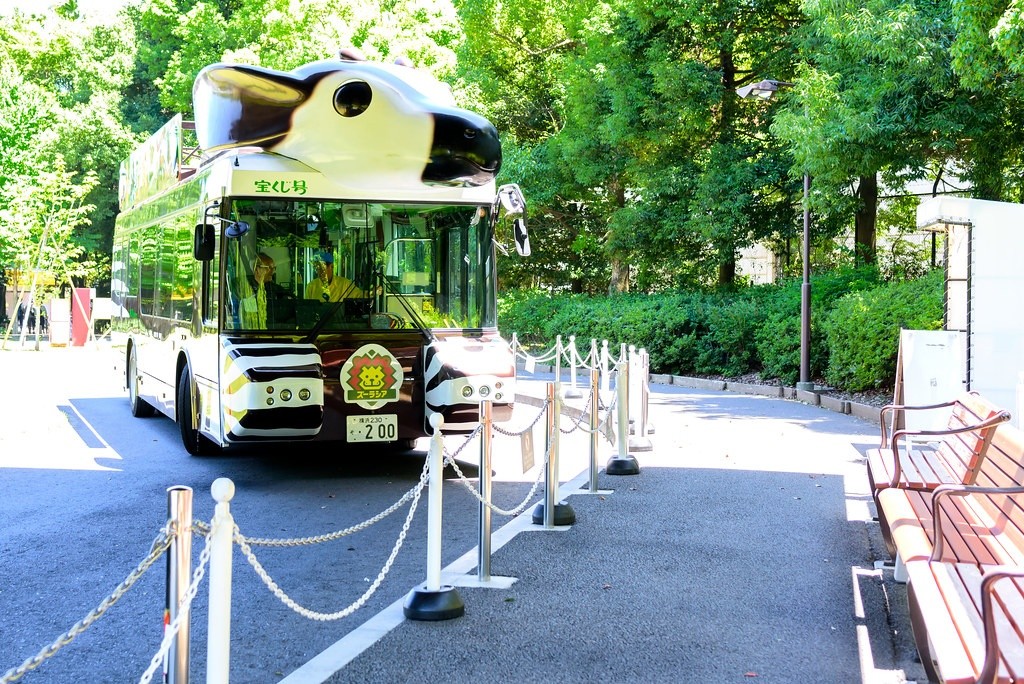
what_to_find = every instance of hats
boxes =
[313,251,334,262]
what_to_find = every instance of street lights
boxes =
[736,78,814,391]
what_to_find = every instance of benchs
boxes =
[866,392,1023,684]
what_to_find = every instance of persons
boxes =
[305,253,383,307]
[230,255,287,313]
[18,297,45,333]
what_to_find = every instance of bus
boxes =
[111,53,532,458]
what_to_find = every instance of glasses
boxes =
[256,264,276,271]
